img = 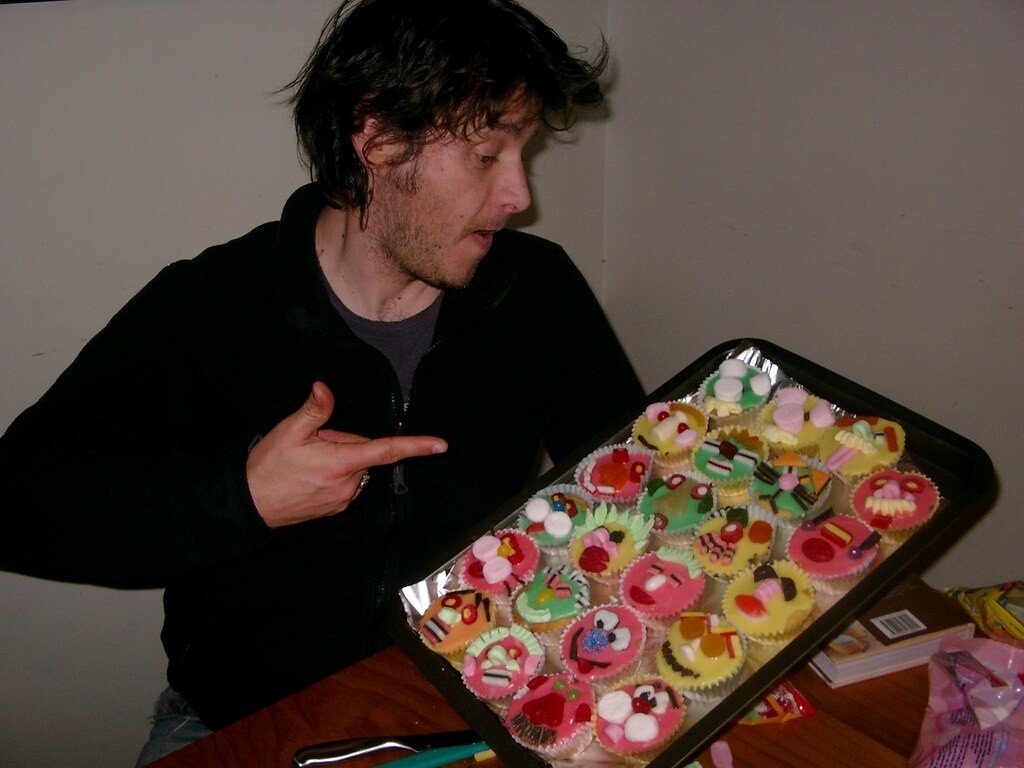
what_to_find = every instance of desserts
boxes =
[417,358,941,763]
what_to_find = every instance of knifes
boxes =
[294,728,482,767]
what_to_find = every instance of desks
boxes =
[136,628,992,768]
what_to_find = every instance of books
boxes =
[809,572,977,691]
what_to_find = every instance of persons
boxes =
[0,0,648,768]
